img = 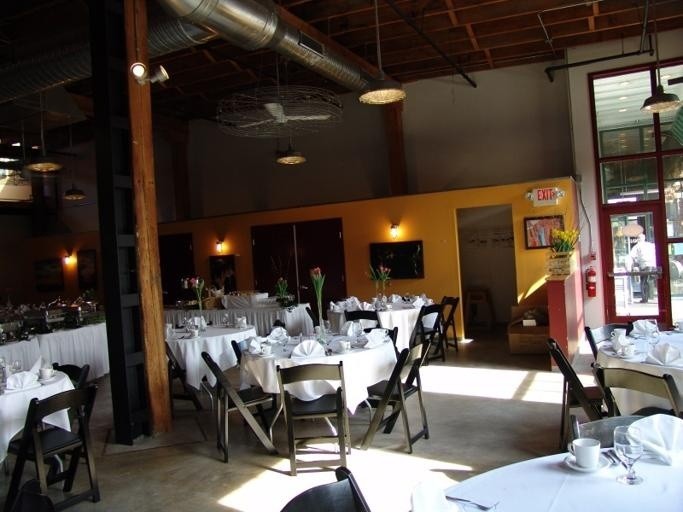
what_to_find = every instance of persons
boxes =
[626,232,658,303]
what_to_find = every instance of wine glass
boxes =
[644,319,660,350]
[404,293,411,304]
[614,425,644,484]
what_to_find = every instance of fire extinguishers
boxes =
[585,265,597,298]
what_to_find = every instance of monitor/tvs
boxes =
[0,144,43,216]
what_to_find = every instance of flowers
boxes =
[377,264,392,294]
[310,267,327,336]
[550,227,579,258]
[275,275,288,304]
[190,277,204,318]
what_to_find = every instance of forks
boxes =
[446,496,501,511]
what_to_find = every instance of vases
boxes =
[548,249,576,277]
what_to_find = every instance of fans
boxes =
[213,60,345,138]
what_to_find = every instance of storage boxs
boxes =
[506,304,549,355]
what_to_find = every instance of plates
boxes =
[564,454,610,472]
[257,352,275,357]
[618,353,641,359]
[674,328,683,333]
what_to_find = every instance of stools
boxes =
[464,288,495,339]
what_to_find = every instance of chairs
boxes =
[199,337,433,476]
[545,318,683,453]
[279,465,371,512]
[431,296,461,356]
[2,383,101,512]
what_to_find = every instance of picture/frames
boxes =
[523,215,565,250]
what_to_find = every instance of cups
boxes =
[621,344,635,355]
[675,320,683,330]
[39,368,55,379]
[611,328,626,337]
[191,330,199,337]
[567,438,601,468]
[426,299,433,304]
[261,345,271,355]
[335,340,346,353]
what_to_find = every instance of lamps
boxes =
[24,92,66,174]
[275,136,306,165]
[358,0,406,106]
[129,61,170,86]
[63,123,86,201]
[639,18,681,114]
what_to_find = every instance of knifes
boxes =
[609,450,628,470]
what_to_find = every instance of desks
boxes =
[164,291,444,443]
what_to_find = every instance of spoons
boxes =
[602,452,619,466]
